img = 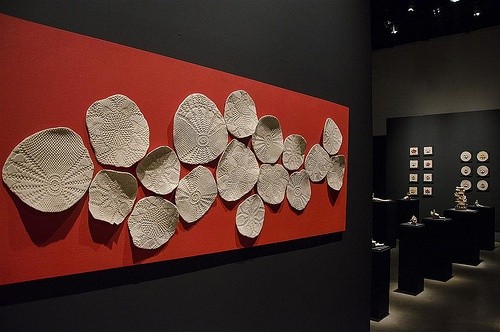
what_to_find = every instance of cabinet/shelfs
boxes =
[369,187,496,323]
